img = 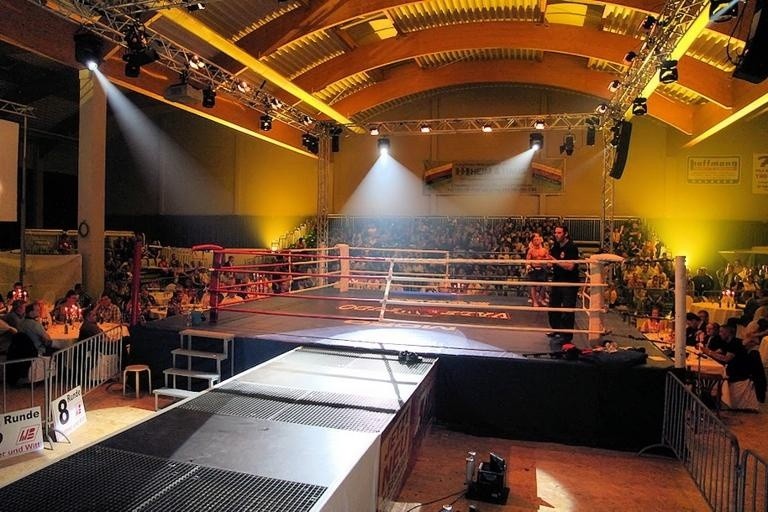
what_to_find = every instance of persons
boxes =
[546,225,579,345]
[525,236,551,307]
[606,220,768,409]
[1,219,315,384]
[327,216,567,298]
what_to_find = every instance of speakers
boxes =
[587,126,595,145]
[732,0,768,84]
[610,120,633,180]
[333,136,339,152]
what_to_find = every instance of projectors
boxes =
[162,83,203,104]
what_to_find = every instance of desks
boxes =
[644,332,726,416]
[689,302,747,324]
[34,320,128,379]
[145,290,215,321]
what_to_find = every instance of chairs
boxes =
[722,350,768,411]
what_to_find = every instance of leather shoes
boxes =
[546,331,570,344]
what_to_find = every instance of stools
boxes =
[122,364,153,399]
